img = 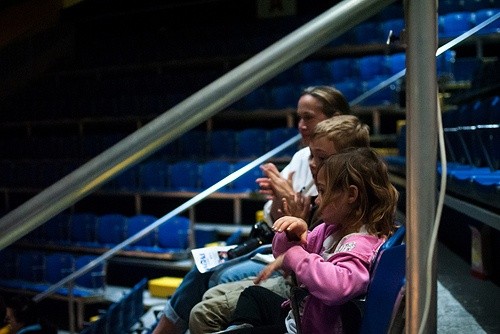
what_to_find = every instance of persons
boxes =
[152,87,350,333]
[189,115,368,334]
[218,149,399,334]
[5,297,57,334]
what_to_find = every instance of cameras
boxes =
[227,221,275,260]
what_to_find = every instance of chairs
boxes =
[0,0,500,334]
